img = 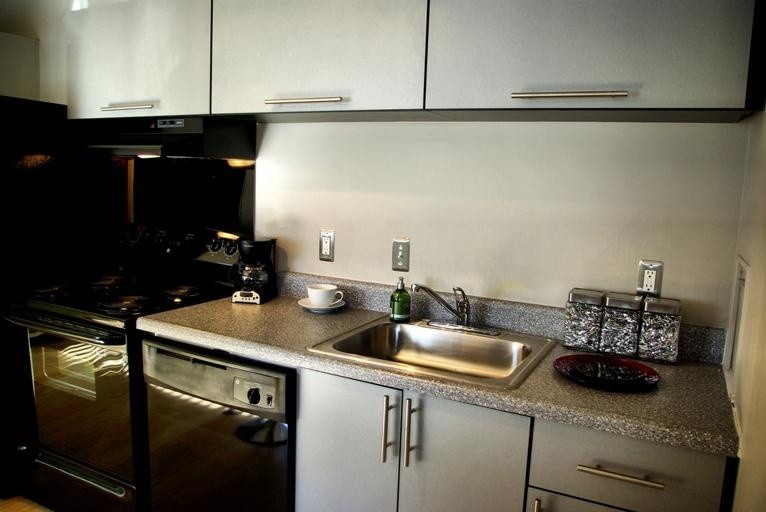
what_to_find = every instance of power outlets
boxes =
[318,229,335,262]
[635,260,664,296]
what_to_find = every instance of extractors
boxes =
[64,118,255,169]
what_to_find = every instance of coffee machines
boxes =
[231,236,280,304]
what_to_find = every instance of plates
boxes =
[552,351,662,393]
[298,297,347,315]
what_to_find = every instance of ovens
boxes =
[0,302,141,512]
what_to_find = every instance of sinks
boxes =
[305,314,559,392]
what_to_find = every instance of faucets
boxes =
[409,283,472,326]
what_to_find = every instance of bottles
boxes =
[389,276,411,324]
[563,286,683,366]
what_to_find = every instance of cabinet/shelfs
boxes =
[208,1,454,123]
[421,0,764,120]
[293,365,535,512]
[520,411,731,512]
[64,1,212,119]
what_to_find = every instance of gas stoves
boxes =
[10,256,233,328]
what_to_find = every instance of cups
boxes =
[305,281,343,306]
